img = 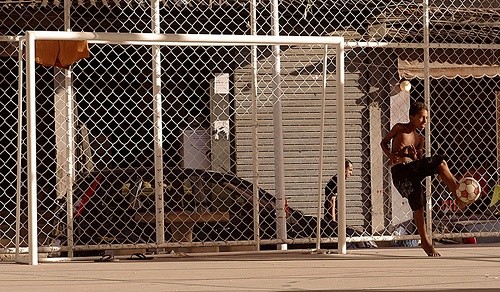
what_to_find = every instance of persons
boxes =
[324,160,353,221]
[380,102,473,257]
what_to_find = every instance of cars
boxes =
[48,166,378,257]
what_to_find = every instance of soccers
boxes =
[455,177,482,203]
